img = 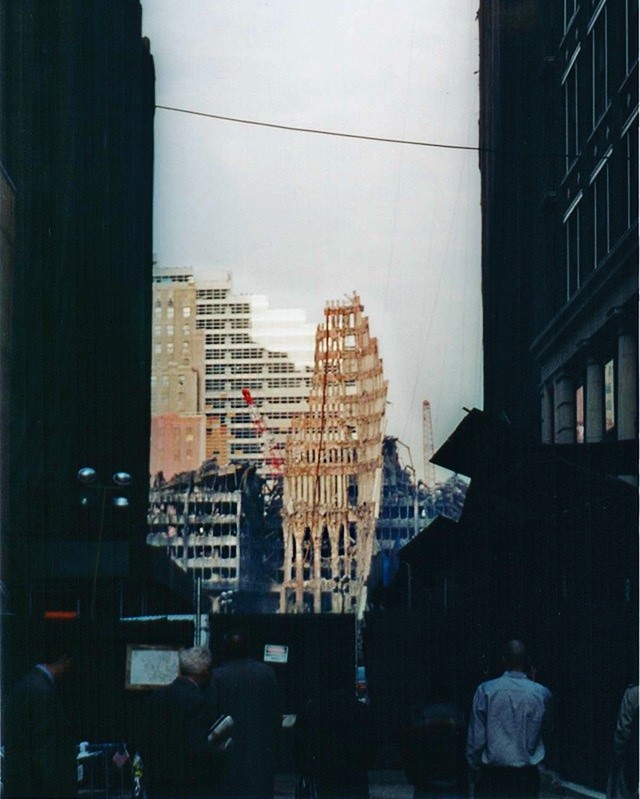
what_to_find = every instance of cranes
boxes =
[241,388,285,484]
[421,400,437,496]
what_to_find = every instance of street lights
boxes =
[77,468,131,627]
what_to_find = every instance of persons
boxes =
[0,631,79,799]
[403,680,472,799]
[606,684,638,799]
[466,640,558,799]
[289,664,372,799]
[138,627,284,799]
